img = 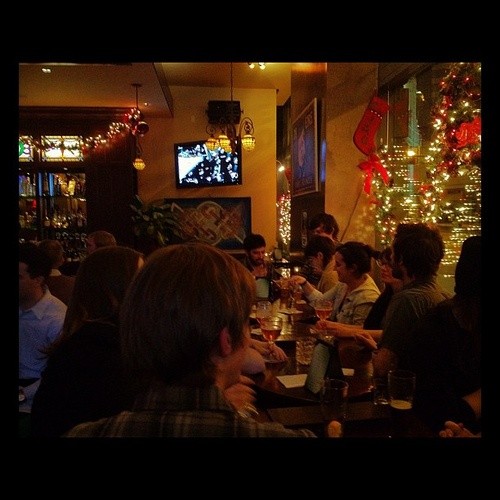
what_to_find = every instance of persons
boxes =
[239,233,272,279]
[19,223,166,446]
[61,243,318,437]
[288,212,482,439]
[241,338,288,374]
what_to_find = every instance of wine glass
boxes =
[261,315,282,362]
[314,297,332,340]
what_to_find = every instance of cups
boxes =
[256,301,272,324]
[320,379,349,421]
[373,378,390,406]
[389,370,416,410]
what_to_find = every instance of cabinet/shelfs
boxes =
[19,104,138,272]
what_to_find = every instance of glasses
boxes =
[376,260,393,273]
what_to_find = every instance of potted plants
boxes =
[132,200,173,254]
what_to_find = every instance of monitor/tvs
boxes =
[174,140,243,188]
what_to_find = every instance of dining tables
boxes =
[249,319,377,400]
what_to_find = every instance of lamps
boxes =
[203,63,255,153]
[128,82,148,170]
[248,62,266,70]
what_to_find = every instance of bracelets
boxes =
[301,281,306,286]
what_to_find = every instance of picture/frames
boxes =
[292,97,318,197]
